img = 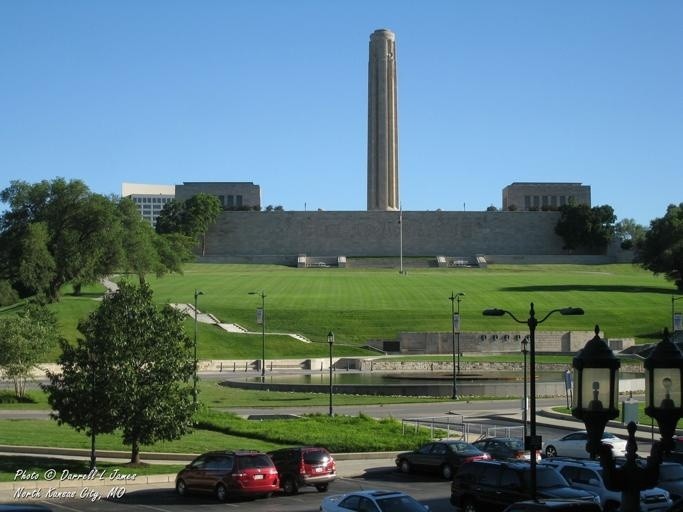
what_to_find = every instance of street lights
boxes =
[482,303,584,500]
[327,330,334,416]
[449,291,464,399]
[571,325,683,512]
[194,289,204,402]
[521,338,529,436]
[248,292,266,375]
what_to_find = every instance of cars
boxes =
[320,490,429,512]
[395,431,683,512]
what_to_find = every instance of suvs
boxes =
[176,447,336,503]
